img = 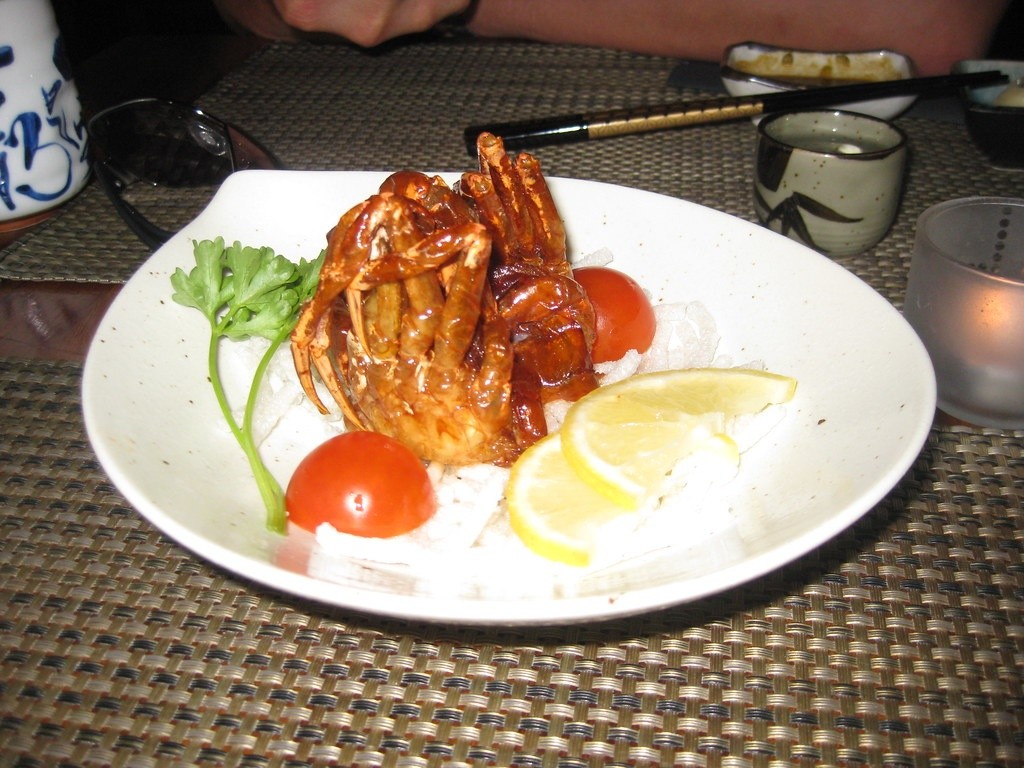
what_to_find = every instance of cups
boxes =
[905,194,1023,429]
[754,110,907,260]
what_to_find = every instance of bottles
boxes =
[0,0,86,223]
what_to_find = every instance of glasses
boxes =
[87,97,281,252]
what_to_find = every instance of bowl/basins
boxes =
[952,57,1024,172]
[718,40,917,121]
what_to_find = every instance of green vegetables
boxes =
[171,236,326,534]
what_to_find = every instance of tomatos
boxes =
[285,430,437,538]
[568,266,655,363]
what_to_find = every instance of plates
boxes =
[79,167,937,629]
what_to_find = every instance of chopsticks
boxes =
[461,70,1010,153]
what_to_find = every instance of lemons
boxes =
[506,430,634,566]
[561,367,798,509]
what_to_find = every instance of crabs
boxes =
[291,130,595,465]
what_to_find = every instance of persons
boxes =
[217,1,1011,87]
